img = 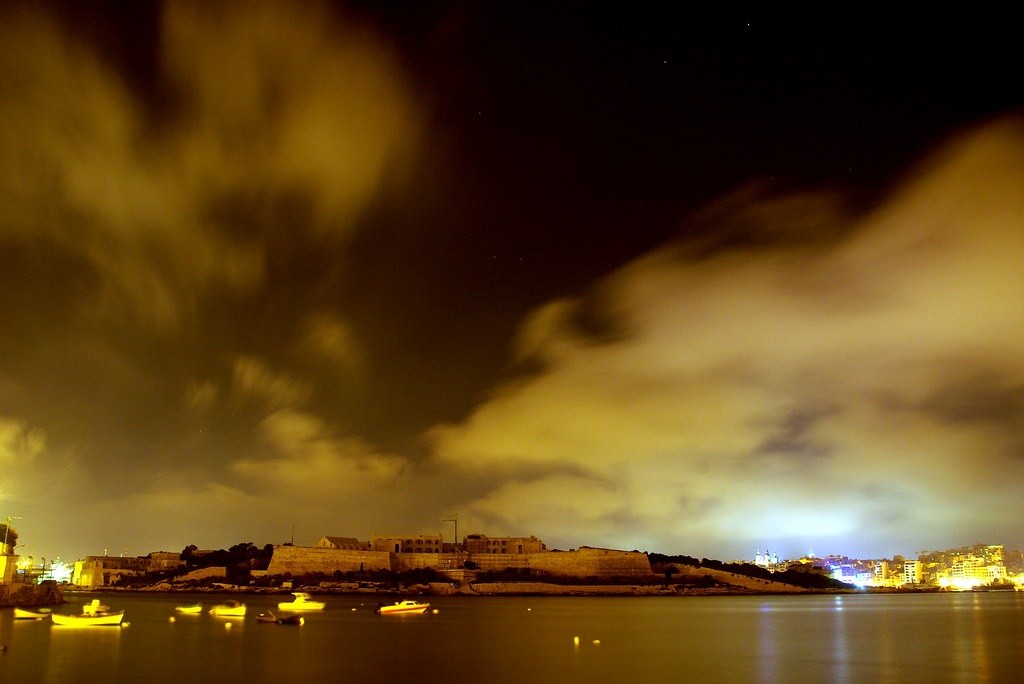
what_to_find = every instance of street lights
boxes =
[50,560,53,579]
[28,556,35,570]
[3,516,23,555]
[42,557,45,580]
[12,543,26,552]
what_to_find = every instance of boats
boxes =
[14,608,51,620]
[83,599,111,615]
[255,611,304,626]
[209,601,247,617]
[51,609,125,625]
[376,598,431,614]
[174,605,205,613]
[276,591,326,611]
